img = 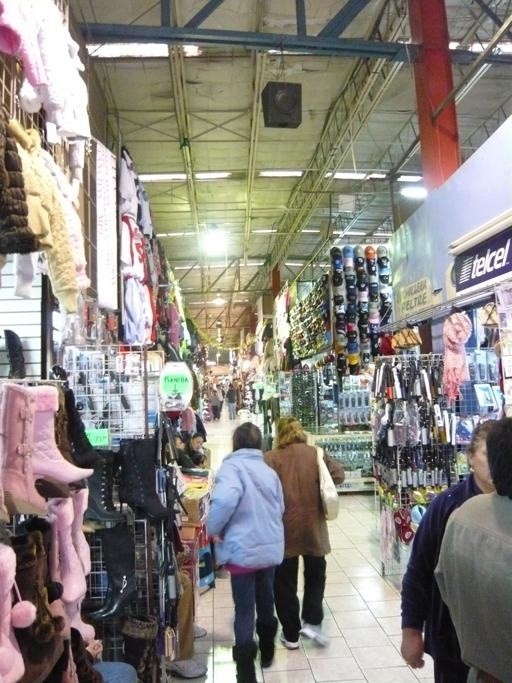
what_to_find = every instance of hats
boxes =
[330,245,393,373]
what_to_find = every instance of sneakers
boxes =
[298,622,330,648]
[166,659,208,679]
[280,632,299,650]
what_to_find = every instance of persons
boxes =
[204,422,287,683]
[260,414,345,650]
[433,417,511,682]
[186,432,207,464]
[211,388,222,419]
[226,383,237,419]
[399,420,499,683]
[166,432,197,466]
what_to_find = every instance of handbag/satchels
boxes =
[391,327,423,350]
[316,446,340,521]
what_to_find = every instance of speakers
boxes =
[262,81,302,129]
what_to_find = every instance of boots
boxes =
[120,615,158,678]
[85,449,123,520]
[0,382,95,683]
[84,524,138,621]
[231,644,258,683]
[119,437,171,520]
[256,616,278,667]
